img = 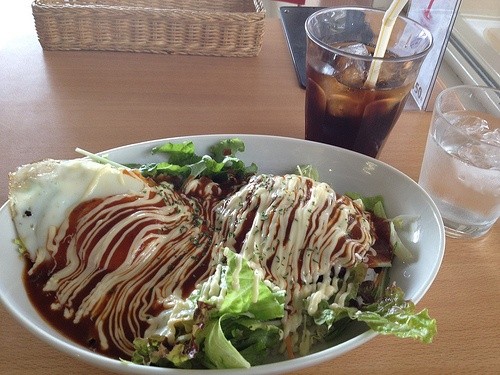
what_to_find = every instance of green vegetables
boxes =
[7,137,438,370]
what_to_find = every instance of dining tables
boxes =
[0,1,500,375]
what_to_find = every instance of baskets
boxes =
[30,1,266,58]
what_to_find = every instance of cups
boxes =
[305,4,434,159]
[418,85,500,239]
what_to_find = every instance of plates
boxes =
[0,134,444,375]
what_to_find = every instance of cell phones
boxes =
[279,6,375,90]
